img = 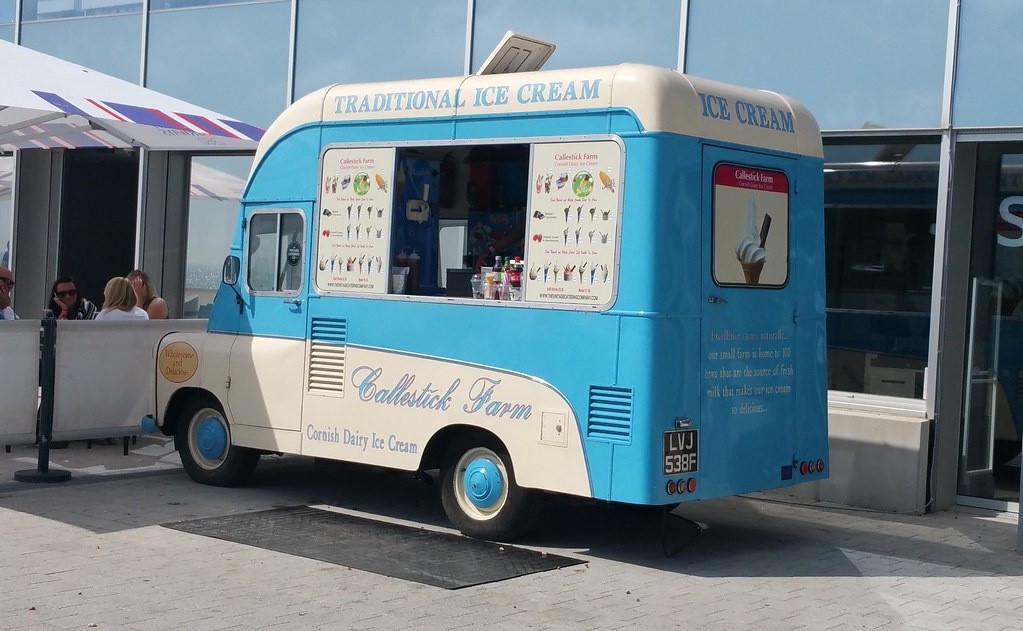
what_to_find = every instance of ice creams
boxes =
[331,203,385,274]
[563,204,611,247]
[529,261,609,285]
[735,191,772,285]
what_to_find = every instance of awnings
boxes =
[0,38,267,151]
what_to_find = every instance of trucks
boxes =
[146,28,828,540]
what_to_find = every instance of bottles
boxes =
[396,247,408,267]
[503,256,511,274]
[520,260,524,270]
[407,250,420,294]
[492,256,502,282]
[510,260,515,269]
[511,256,522,287]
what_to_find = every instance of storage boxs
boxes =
[447,268,481,298]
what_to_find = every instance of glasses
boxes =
[0,276,15,286]
[55,287,78,297]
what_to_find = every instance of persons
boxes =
[126,270,170,319]
[48,276,97,320]
[95,277,149,320]
[0,265,20,320]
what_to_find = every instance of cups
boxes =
[392,266,410,294]
[470,273,483,299]
[485,273,494,299]
